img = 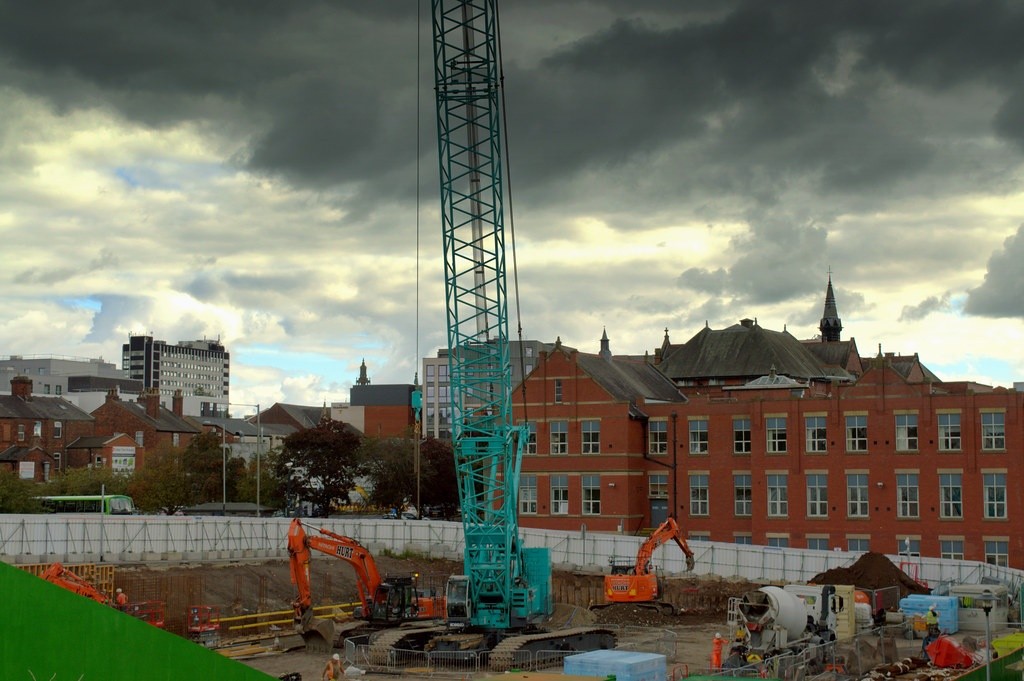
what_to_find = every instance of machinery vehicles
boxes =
[369,0,620,674]
[603,517,697,617]
[286,518,448,646]
[38,563,167,630]
[726,584,839,670]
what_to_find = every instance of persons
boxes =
[921,605,942,635]
[116,587,128,611]
[322,653,344,681]
[712,626,755,673]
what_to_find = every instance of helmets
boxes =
[333,653,340,660]
[929,606,934,610]
[116,588,122,592]
[715,633,721,638]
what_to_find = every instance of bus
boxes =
[29,496,138,516]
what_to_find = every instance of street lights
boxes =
[203,420,228,516]
[982,590,992,681]
